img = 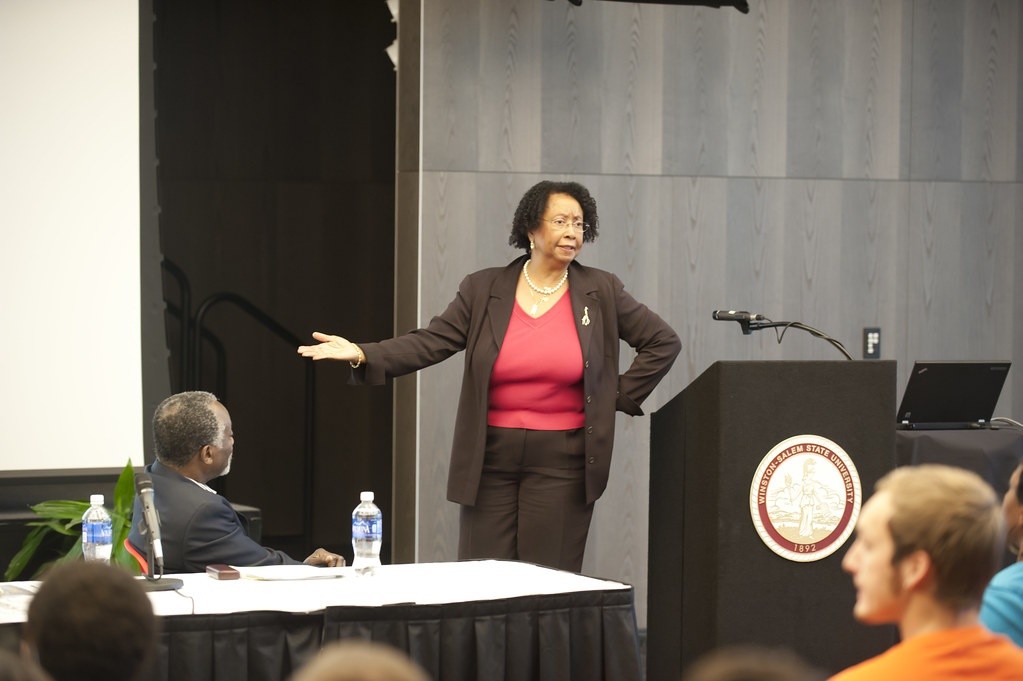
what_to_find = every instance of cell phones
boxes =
[207,565,239,580]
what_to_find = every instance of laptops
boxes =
[896,359,1013,430]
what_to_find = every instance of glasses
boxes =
[543,217,590,233]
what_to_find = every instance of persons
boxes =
[0,562,157,681]
[683,643,821,681]
[286,638,431,681]
[827,465,1023,681]
[298,181,682,574]
[128,390,346,575]
[977,462,1023,647]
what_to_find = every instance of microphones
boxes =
[712,310,765,321]
[134,473,164,567]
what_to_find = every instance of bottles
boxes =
[349,490,384,580]
[81,493,113,571]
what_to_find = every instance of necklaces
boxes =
[523,259,568,317]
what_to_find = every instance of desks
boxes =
[0,556,645,681]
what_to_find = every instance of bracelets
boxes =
[350,344,361,368]
